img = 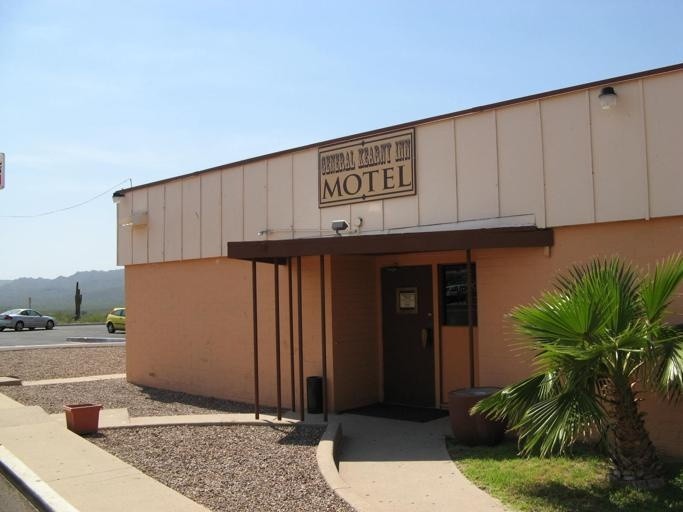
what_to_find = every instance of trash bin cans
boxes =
[307,377,323,413]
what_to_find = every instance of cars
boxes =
[106,308,125,333]
[0,309,55,331]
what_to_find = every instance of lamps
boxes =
[598,86,618,109]
[257,231,269,236]
[112,191,124,204]
[333,220,348,237]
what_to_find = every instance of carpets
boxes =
[338,401,449,423]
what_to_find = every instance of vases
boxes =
[63,404,104,435]
[448,386,508,448]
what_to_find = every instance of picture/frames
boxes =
[396,287,418,314]
